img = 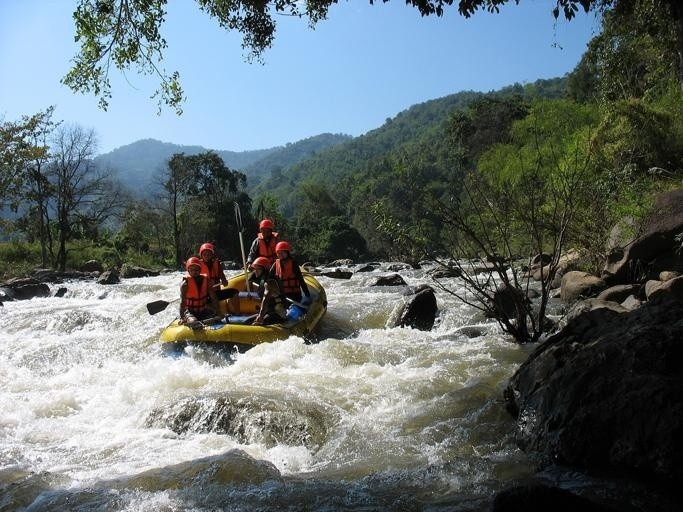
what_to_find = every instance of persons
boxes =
[251,256,288,325]
[246,220,278,271]
[179,257,218,326]
[198,243,238,303]
[268,241,311,307]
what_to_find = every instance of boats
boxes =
[159,265,328,351]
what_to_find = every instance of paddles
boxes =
[147,271,251,315]
[252,283,307,309]
[234,202,251,297]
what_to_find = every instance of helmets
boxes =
[275,241,291,254]
[251,256,271,271]
[185,257,201,270]
[199,243,214,257]
[259,220,275,229]
